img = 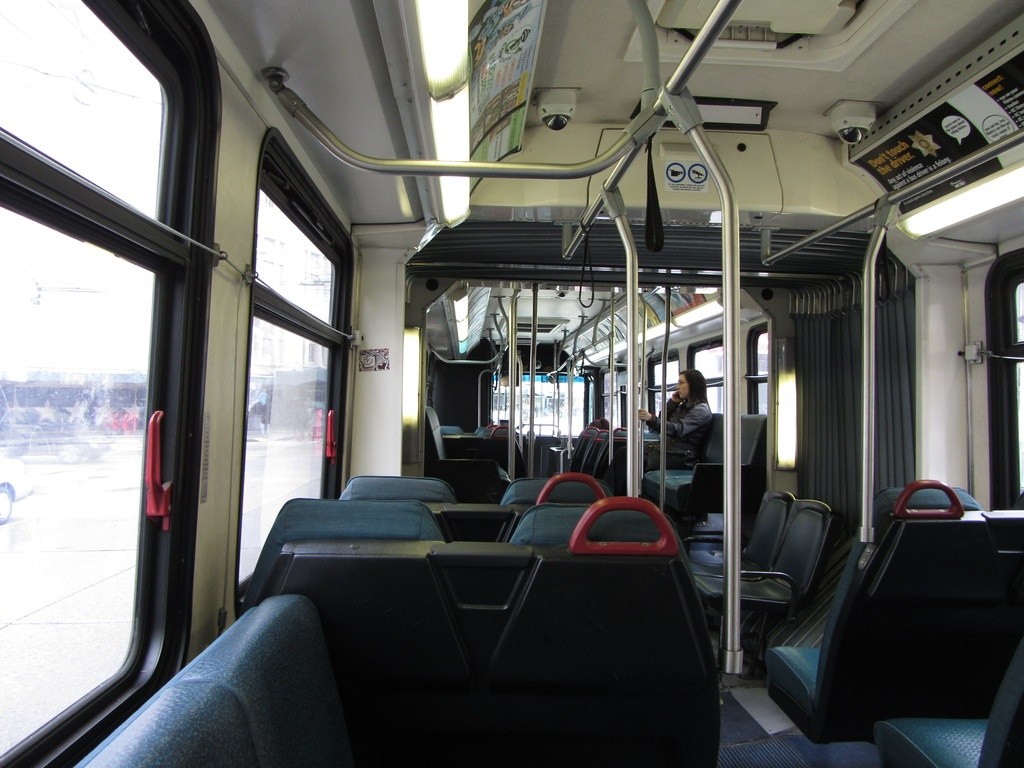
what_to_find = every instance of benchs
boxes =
[75,411,1023,768]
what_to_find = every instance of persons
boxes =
[602,370,714,498]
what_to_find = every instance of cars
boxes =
[0,456,26,525]
[0,407,112,467]
